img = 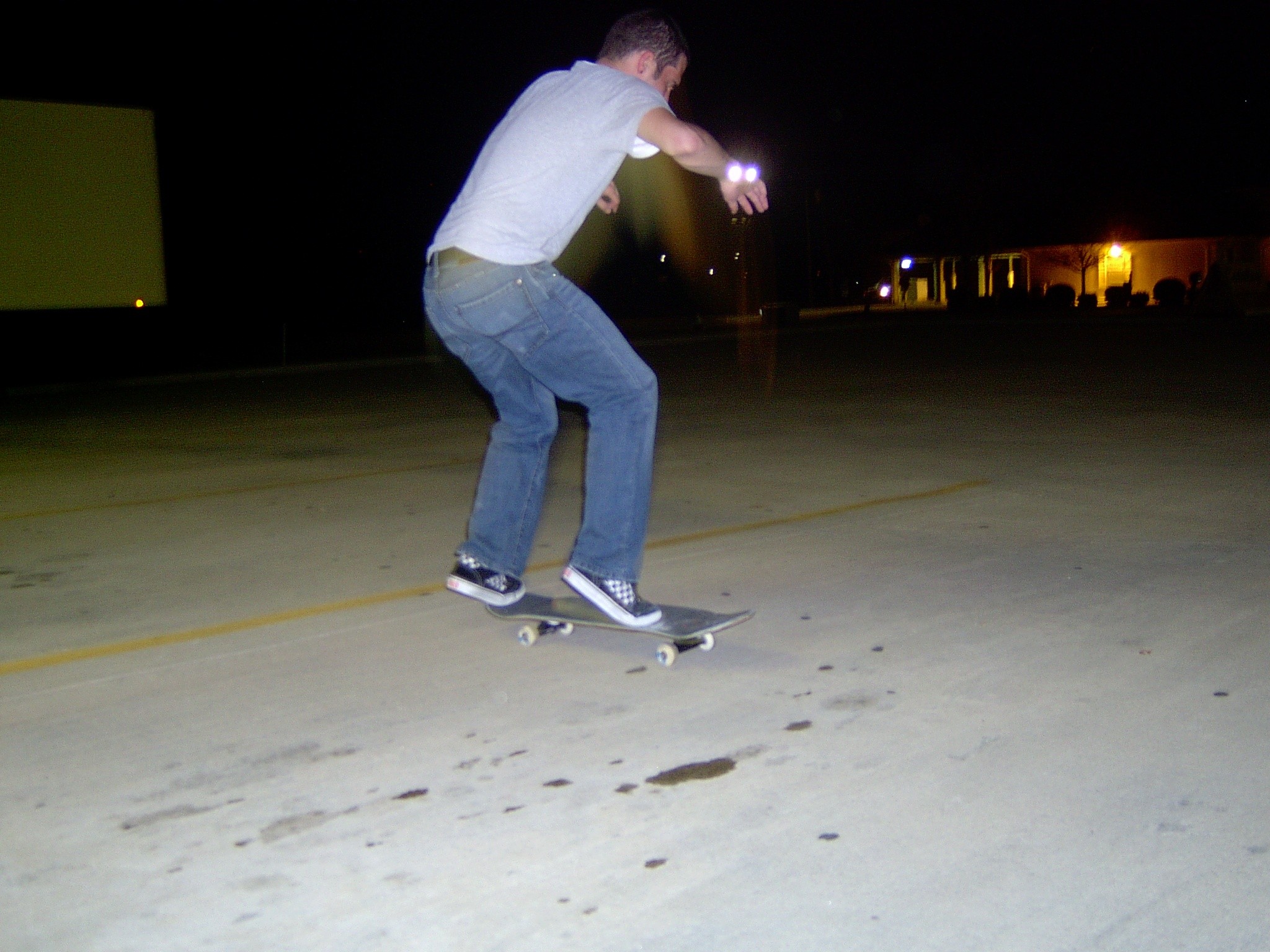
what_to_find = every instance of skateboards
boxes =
[484,587,756,668]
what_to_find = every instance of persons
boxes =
[423,9,769,627]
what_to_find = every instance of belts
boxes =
[430,248,483,265]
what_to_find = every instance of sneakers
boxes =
[446,555,525,607]
[561,564,661,626]
